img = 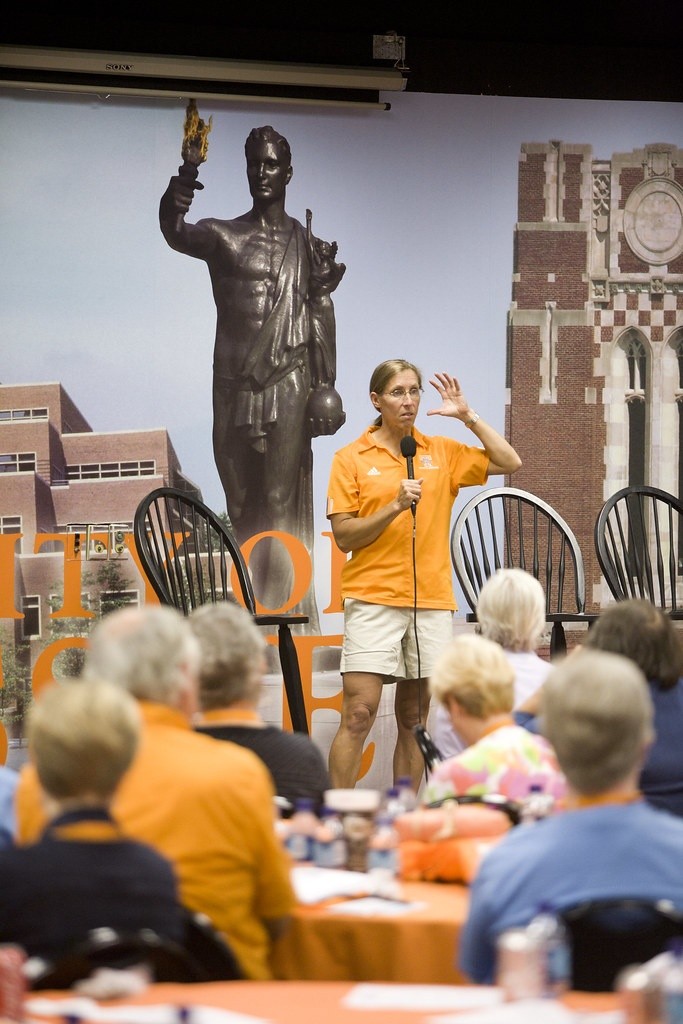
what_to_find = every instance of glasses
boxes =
[381,388,425,398]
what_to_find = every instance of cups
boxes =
[498,918,570,1005]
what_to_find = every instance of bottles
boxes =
[276,777,420,886]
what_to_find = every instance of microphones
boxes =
[400,436,416,518]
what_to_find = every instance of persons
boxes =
[325,359,521,791]
[158,123,346,634]
[0,568,683,1024]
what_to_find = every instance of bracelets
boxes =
[465,414,482,428]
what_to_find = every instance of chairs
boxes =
[451,486,601,665]
[595,486,682,620]
[134,487,309,733]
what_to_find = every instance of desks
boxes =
[20,872,658,1024]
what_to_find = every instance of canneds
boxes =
[496,930,547,1000]
